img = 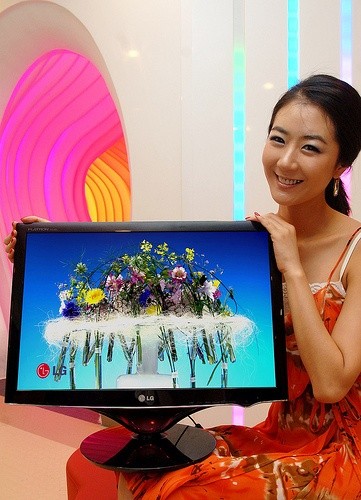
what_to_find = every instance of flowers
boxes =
[56,239,238,319]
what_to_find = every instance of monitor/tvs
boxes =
[4,221,288,404]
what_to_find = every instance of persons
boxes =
[4,74,360,500]
